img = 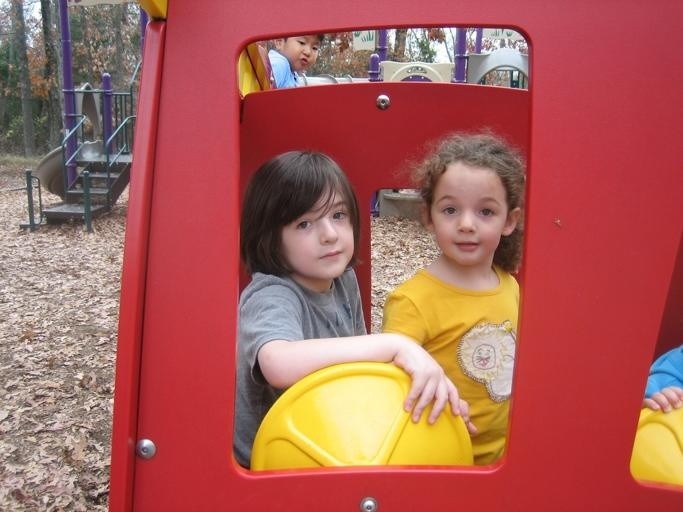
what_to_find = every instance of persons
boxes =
[267,33,325,89]
[639,342,682,414]
[383,129,520,467]
[236,151,478,472]
